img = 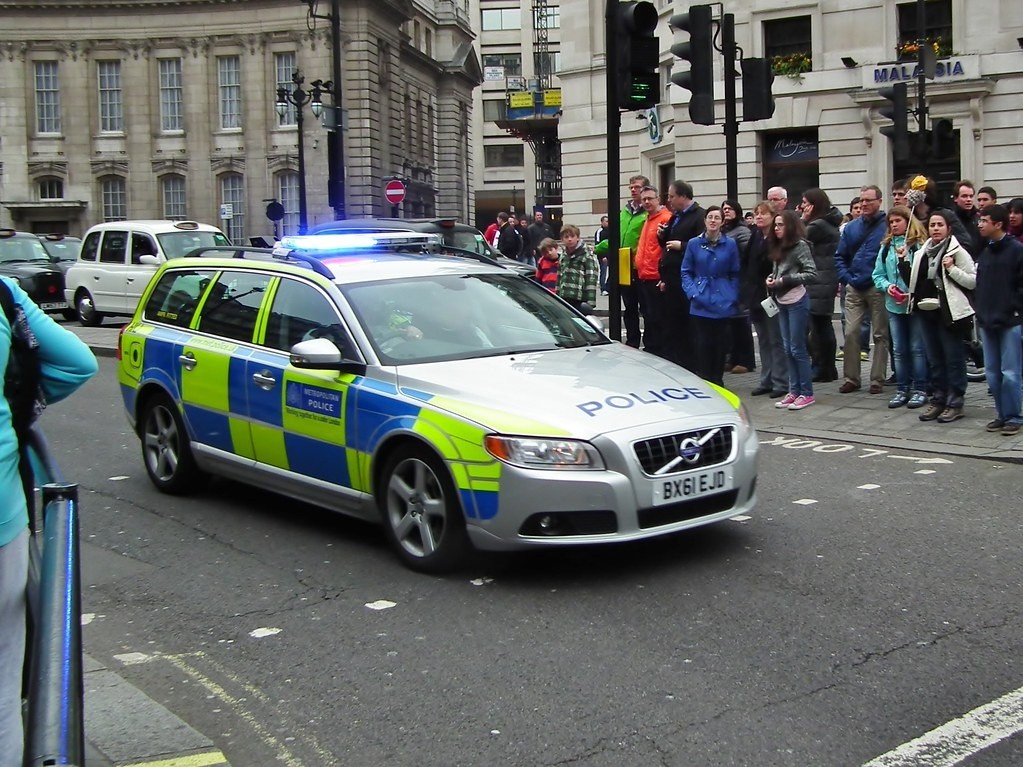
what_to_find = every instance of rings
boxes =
[416,335,420,338]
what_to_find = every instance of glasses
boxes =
[858,198,879,204]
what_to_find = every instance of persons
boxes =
[297,289,424,363]
[0,277,99,767]
[485,174,1023,438]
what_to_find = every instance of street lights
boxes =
[274,67,334,237]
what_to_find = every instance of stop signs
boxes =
[384,180,406,204]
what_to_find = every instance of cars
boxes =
[63,220,235,326]
[117,228,761,577]
[34,233,81,277]
[0,232,78,322]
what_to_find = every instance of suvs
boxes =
[311,217,537,292]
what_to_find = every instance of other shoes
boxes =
[1000,424,1020,435]
[769,391,786,398]
[731,365,753,373]
[600,291,608,296]
[869,384,880,394]
[835,350,844,361]
[839,381,861,393]
[751,388,774,396]
[861,352,869,361]
[986,419,1003,432]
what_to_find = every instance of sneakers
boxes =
[919,405,944,420]
[888,390,910,408]
[775,391,815,410]
[937,406,965,422]
[907,391,928,408]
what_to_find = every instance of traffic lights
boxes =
[670,5,714,125]
[879,82,909,161]
[932,118,954,158]
[613,1,661,110]
